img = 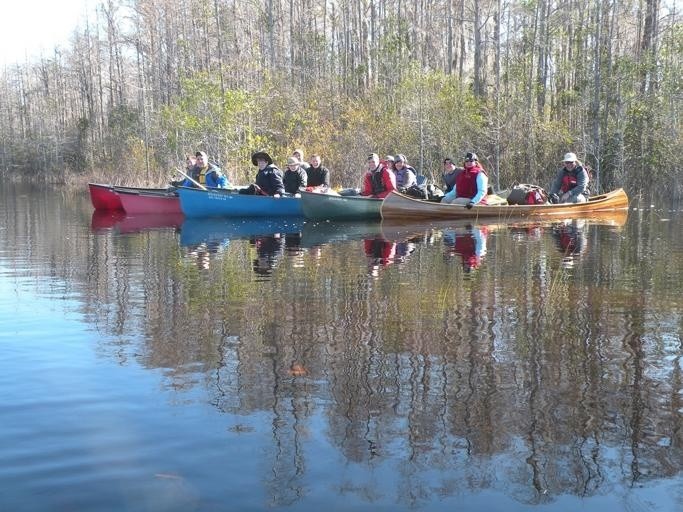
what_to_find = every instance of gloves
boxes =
[465,202,475,210]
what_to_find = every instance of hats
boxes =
[383,154,407,165]
[195,151,206,158]
[252,150,272,166]
[561,152,577,162]
[444,158,455,165]
[464,152,479,163]
[287,148,304,166]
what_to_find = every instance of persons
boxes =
[381,155,395,173]
[443,158,464,194]
[360,153,396,200]
[281,156,306,199]
[548,153,590,204]
[291,150,311,169]
[305,154,329,193]
[393,154,417,191]
[166,150,229,197]
[238,150,285,200]
[168,155,194,187]
[439,152,488,210]
